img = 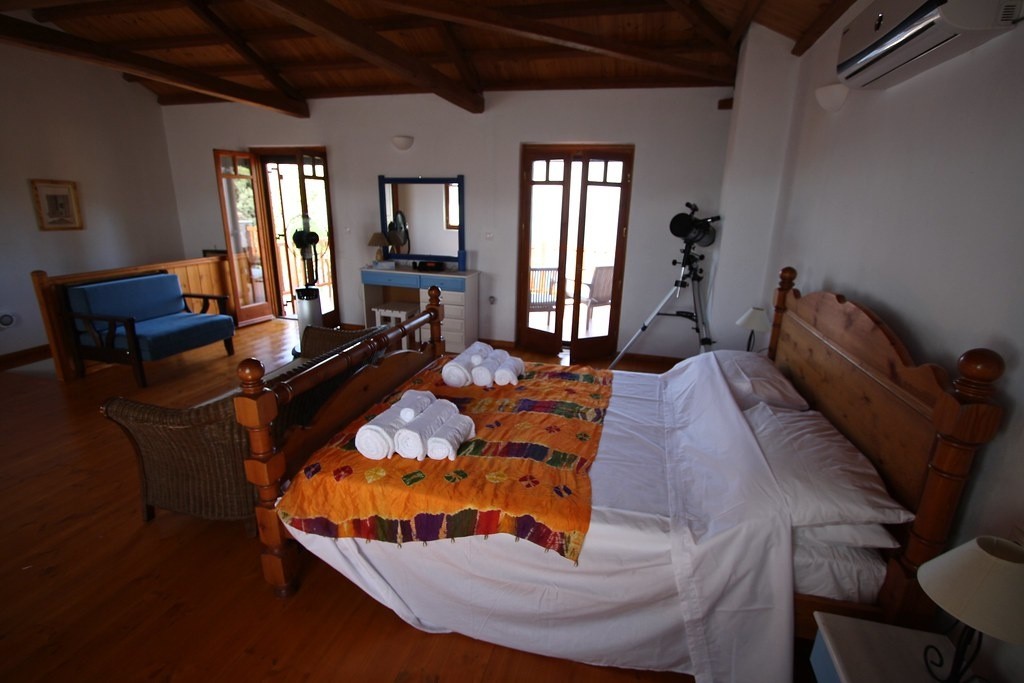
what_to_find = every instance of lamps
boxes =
[367,233,389,262]
[735,307,771,351]
[916,535,1024,683]
[387,231,403,254]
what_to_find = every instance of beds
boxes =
[233,266,1008,642]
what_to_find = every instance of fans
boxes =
[394,210,411,254]
[285,215,329,299]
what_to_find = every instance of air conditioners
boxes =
[836,0,1024,91]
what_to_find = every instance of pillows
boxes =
[744,402,916,527]
[791,522,900,549]
[714,350,810,412]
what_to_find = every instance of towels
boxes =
[355,388,476,462]
[441,341,527,388]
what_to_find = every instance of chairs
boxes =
[529,266,614,330]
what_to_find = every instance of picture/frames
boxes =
[30,178,84,232]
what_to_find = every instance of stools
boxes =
[370,301,419,350]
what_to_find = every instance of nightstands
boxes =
[809,612,981,683]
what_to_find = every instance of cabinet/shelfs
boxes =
[360,264,479,355]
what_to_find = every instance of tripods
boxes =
[607,265,710,370]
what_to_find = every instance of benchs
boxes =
[54,270,236,389]
[100,324,390,537]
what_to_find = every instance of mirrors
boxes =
[377,174,465,262]
